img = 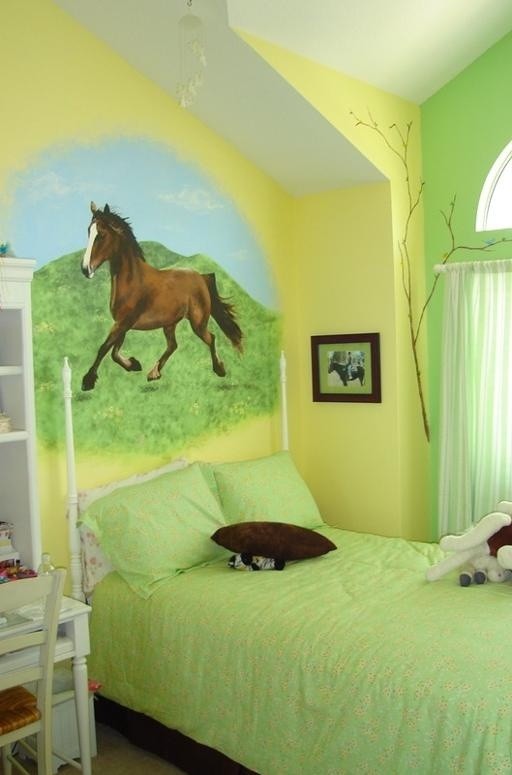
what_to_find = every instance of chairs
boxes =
[0,565,67,775]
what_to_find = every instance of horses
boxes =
[328,359,365,386]
[81,201,244,391]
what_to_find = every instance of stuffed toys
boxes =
[229,553,285,572]
[425,499,512,587]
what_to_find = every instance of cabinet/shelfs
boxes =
[0,255,42,584]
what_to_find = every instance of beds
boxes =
[74,449,510,775]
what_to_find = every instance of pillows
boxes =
[83,454,327,599]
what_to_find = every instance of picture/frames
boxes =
[308,330,382,401]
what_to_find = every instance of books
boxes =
[0,412,12,436]
[0,520,82,656]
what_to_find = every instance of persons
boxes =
[344,352,353,380]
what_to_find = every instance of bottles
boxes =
[37,552,54,576]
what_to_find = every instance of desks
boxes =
[0,593,92,775]
